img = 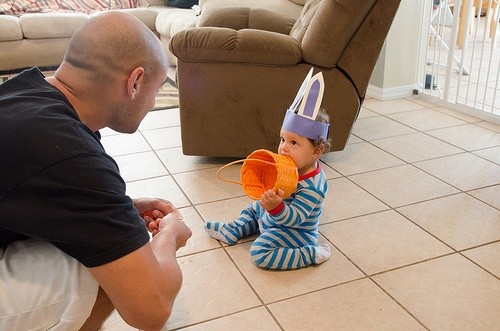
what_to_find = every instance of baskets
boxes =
[217,148,298,200]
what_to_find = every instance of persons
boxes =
[0,11,192,331]
[204,107,331,270]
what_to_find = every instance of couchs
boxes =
[0,0,306,88]
[169,0,400,157]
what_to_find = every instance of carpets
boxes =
[0,70,179,111]
